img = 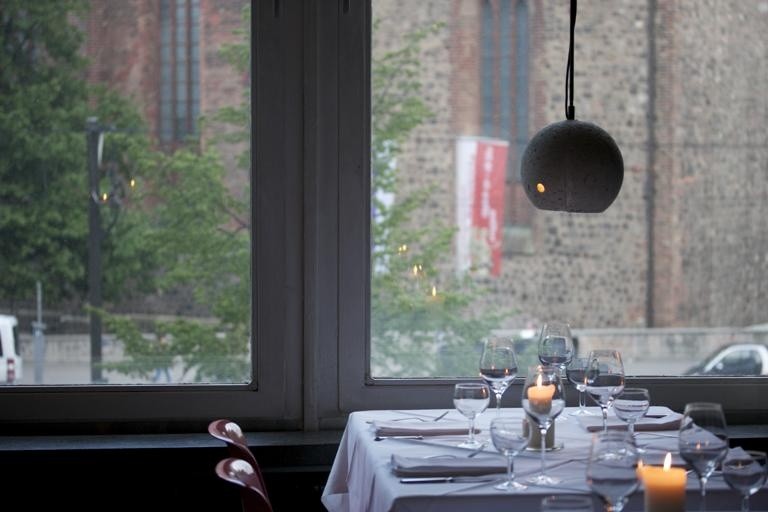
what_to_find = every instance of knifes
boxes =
[399,475,496,487]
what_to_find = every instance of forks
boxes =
[424,443,485,461]
[364,411,449,425]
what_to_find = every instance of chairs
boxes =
[202,413,290,511]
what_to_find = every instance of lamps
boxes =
[518,0,625,215]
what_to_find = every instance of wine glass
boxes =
[681,400,727,512]
[722,451,768,511]
[449,320,652,511]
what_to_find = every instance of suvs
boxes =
[690,345,768,378]
[0,317,24,386]
